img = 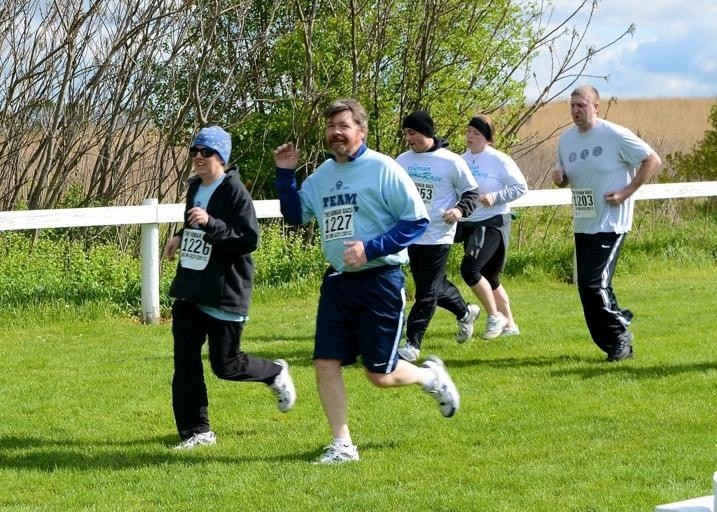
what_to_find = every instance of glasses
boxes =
[188,147,217,157]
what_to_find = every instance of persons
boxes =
[455,115,528,339]
[274,99,459,465]
[550,86,662,362]
[394,111,481,363]
[164,126,296,452]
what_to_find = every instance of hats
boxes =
[193,126,232,166]
[402,111,433,137]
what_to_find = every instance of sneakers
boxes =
[397,341,420,362]
[483,312,520,339]
[173,430,217,449]
[457,304,481,344]
[422,355,460,417]
[606,329,634,361]
[265,359,296,413]
[311,443,360,466]
